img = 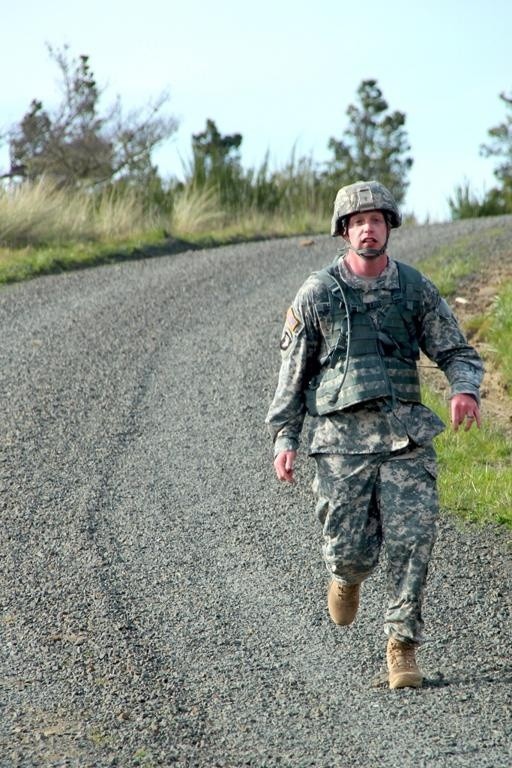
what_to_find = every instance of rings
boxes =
[468,414,476,422]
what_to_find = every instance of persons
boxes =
[260,182,486,692]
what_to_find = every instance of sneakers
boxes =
[387,638,423,688]
[328,579,359,625]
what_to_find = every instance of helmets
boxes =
[331,181,401,237]
